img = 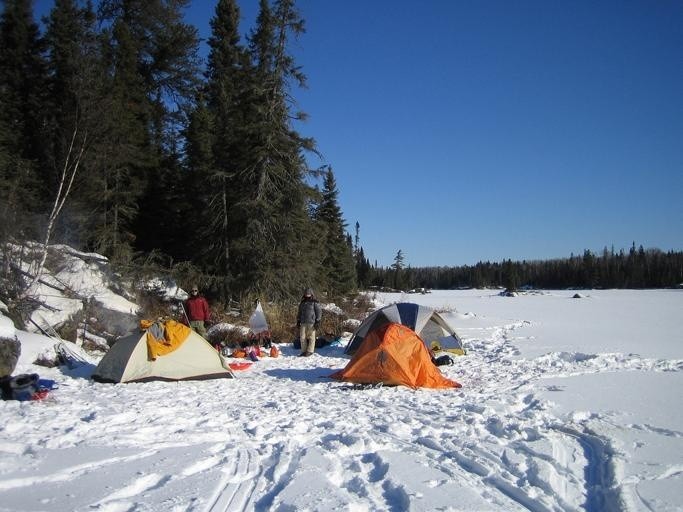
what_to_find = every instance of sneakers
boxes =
[298,351,313,356]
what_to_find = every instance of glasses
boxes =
[191,289,198,293]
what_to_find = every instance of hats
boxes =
[304,288,315,296]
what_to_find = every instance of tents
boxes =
[91,318,236,383]
[327,322,463,391]
[341,302,467,356]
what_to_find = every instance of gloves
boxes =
[297,319,302,329]
[204,321,211,328]
[314,320,320,329]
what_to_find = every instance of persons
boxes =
[182,284,211,338]
[296,285,322,356]
